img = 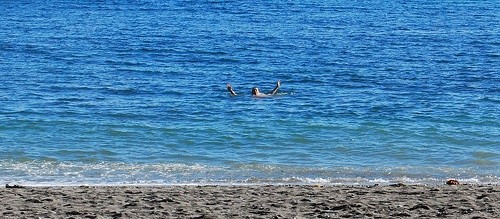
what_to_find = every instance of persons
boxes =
[225,80,281,96]
